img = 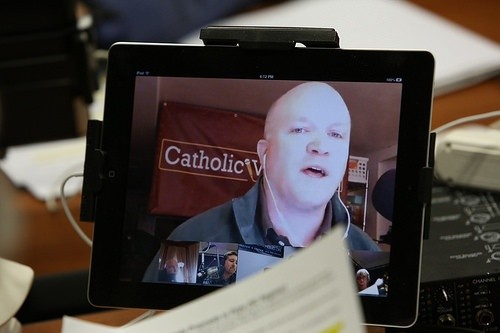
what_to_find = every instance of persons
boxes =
[165,257,179,275]
[207,250,237,286]
[143,82,384,296]
[355,267,386,295]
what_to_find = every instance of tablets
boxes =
[87,40,434,329]
